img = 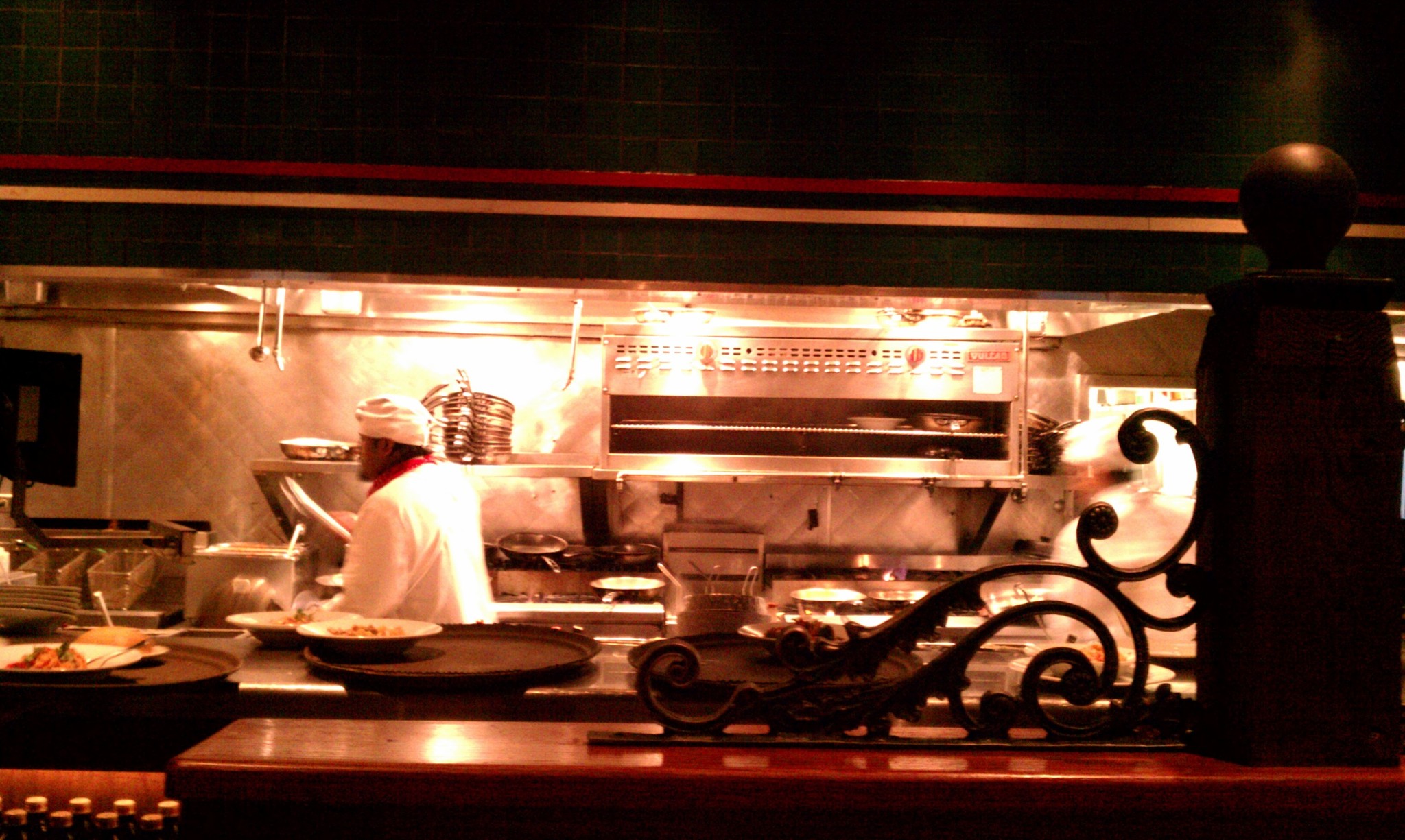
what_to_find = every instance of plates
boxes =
[848,416,907,430]
[0,642,143,679]
[226,610,362,647]
[0,547,81,636]
[297,619,443,654]
[142,644,171,658]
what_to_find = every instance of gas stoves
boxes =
[489,558,665,595]
[770,579,987,618]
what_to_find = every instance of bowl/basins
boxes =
[916,413,984,433]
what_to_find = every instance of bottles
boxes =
[0,795,182,840]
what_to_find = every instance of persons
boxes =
[315,393,504,625]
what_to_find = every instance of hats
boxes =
[1064,410,1146,472]
[356,394,430,450]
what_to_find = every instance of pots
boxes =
[563,543,661,567]
[868,590,930,610]
[589,576,665,602]
[789,588,866,610]
[498,531,569,573]
[276,366,516,465]
